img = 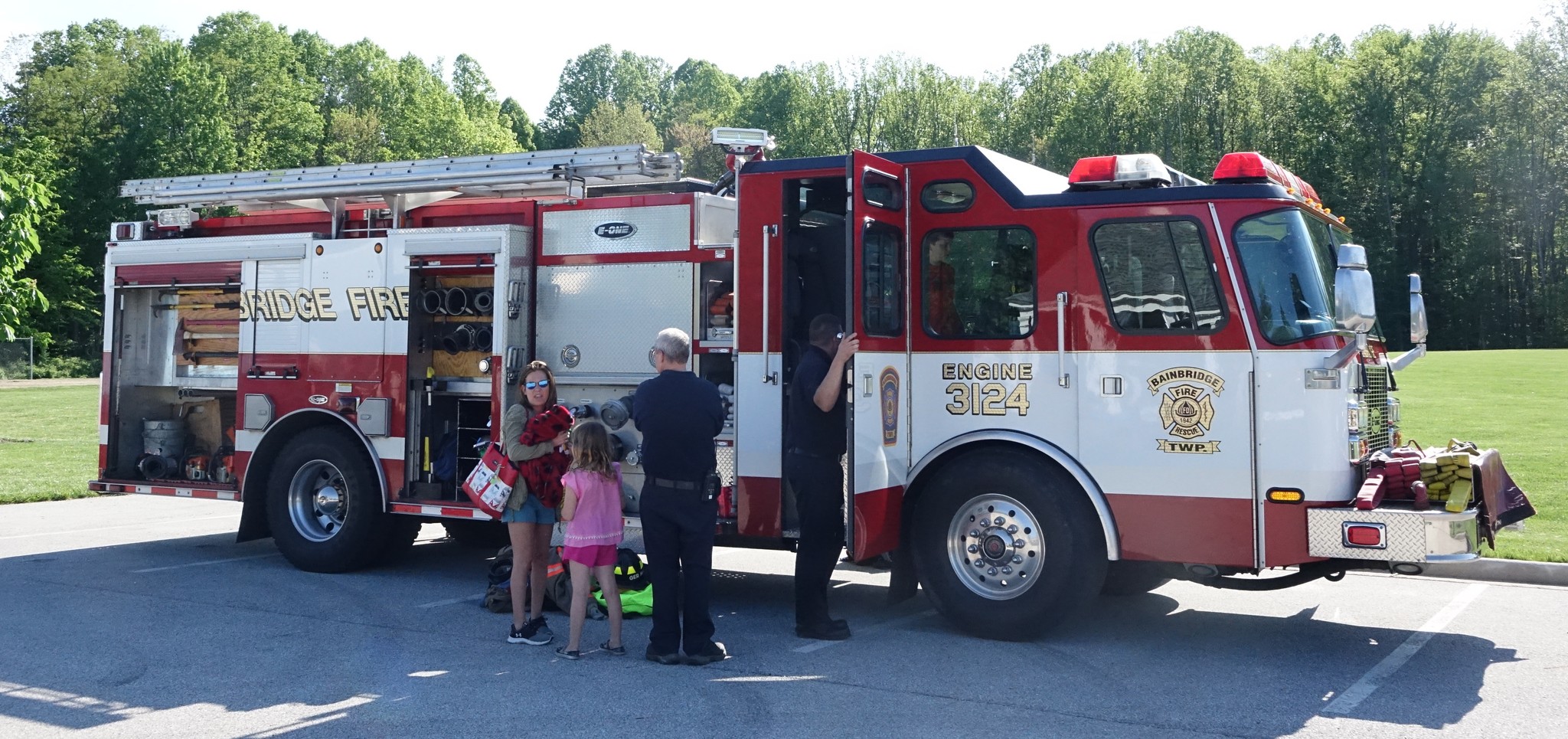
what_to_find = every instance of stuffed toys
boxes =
[519,405,576,508]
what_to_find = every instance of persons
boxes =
[780,314,859,640]
[502,361,573,645]
[1116,234,1175,329]
[555,422,625,659]
[928,231,964,336]
[633,328,727,664]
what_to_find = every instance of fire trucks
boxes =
[88,125,1533,646]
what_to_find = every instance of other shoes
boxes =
[797,619,849,639]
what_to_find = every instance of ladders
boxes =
[120,145,682,202]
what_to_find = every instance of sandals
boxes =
[556,646,580,659]
[599,639,625,655]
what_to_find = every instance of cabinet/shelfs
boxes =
[454,398,493,503]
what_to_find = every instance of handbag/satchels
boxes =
[461,403,528,519]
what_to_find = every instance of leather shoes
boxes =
[646,643,679,664]
[687,642,726,665]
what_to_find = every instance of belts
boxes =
[644,476,703,490]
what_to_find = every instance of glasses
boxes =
[525,380,550,390]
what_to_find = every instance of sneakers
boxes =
[529,615,552,635]
[507,622,553,645]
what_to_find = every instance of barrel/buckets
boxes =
[144,419,184,458]
